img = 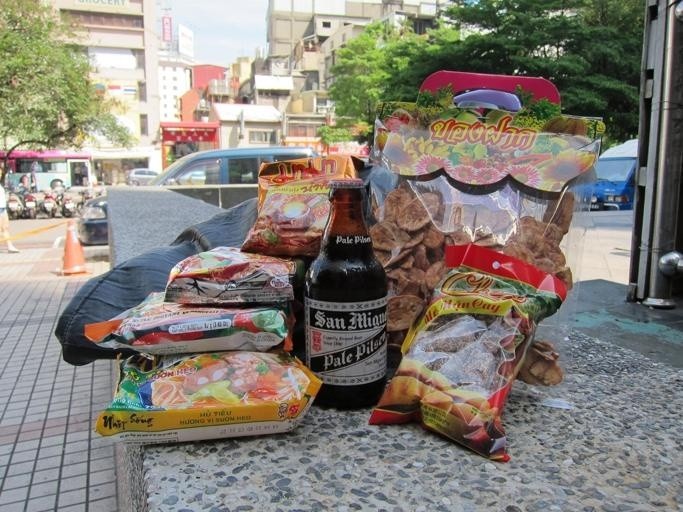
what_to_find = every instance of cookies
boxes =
[370,181,574,387]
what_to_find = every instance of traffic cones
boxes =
[49,218,93,277]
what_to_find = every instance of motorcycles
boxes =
[4,183,105,218]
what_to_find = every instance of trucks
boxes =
[569,138,638,211]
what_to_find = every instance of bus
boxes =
[0,149,96,192]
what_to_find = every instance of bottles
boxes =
[306,175,388,412]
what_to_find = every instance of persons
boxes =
[19,175,35,191]
[0,168,20,253]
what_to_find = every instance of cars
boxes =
[73,146,319,246]
[124,167,159,188]
